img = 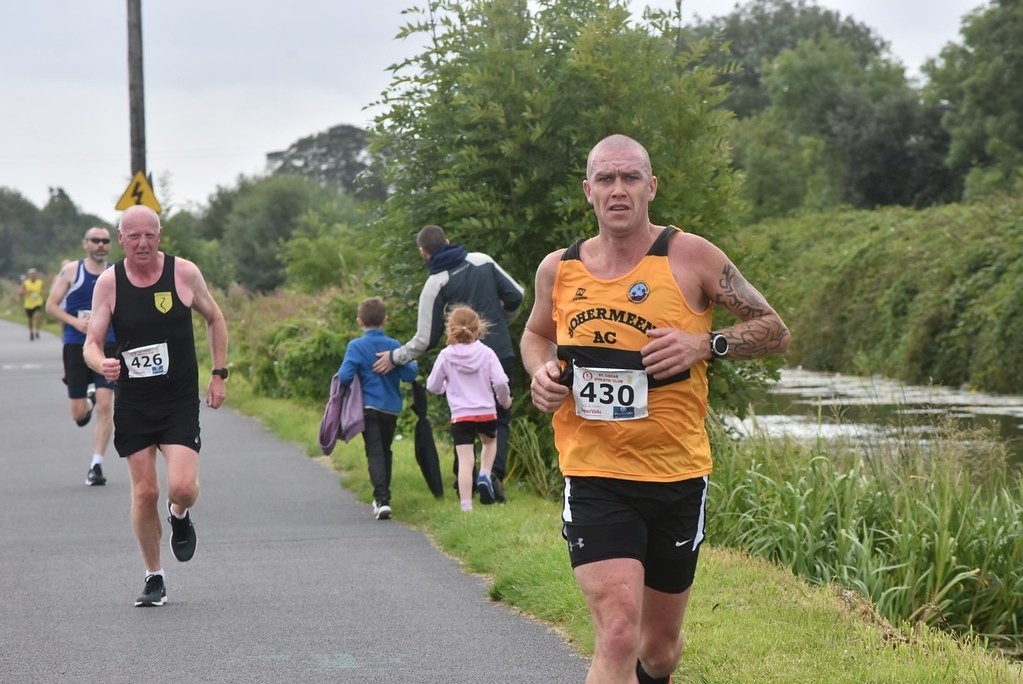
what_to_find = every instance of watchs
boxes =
[212,368,228,379]
[708,329,729,362]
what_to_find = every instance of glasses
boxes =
[86,238,110,244]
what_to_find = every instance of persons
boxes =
[337,296,419,518]
[45,226,119,485]
[82,204,228,607]
[520,133,793,684]
[17,268,48,341]
[372,229,525,504]
[427,305,514,514]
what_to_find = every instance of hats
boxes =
[29,267,37,273]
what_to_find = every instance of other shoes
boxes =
[454,479,475,499]
[490,473,504,502]
[30,332,34,340]
[35,333,39,338]
[371,500,392,520]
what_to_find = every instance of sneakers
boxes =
[77,387,96,426]
[166,498,197,562]
[86,463,106,485]
[477,475,495,504]
[134,574,168,606]
